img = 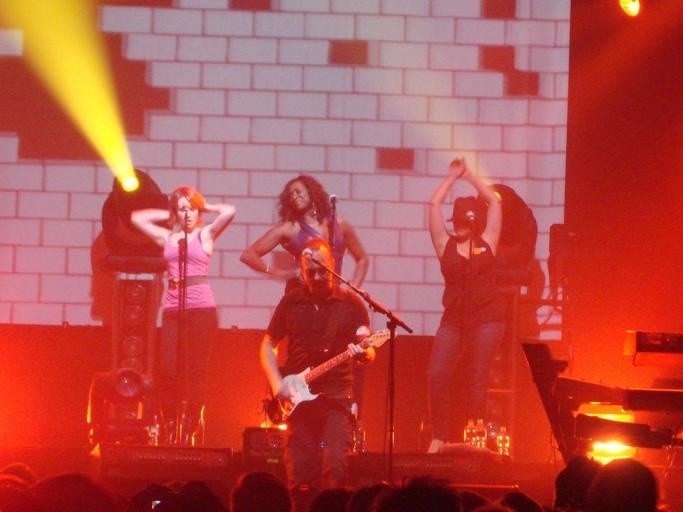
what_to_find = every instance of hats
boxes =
[447,196,481,222]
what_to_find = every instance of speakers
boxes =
[101,447,233,503]
[96,256,159,445]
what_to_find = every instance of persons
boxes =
[128,181,237,448]
[238,172,371,297]
[308,476,545,512]
[553,455,659,512]
[2,463,291,512]
[256,234,379,499]
[425,154,504,455]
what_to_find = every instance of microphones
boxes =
[329,195,337,210]
[467,209,478,224]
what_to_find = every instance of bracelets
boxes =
[265,264,269,274]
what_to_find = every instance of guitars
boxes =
[266,330,397,424]
